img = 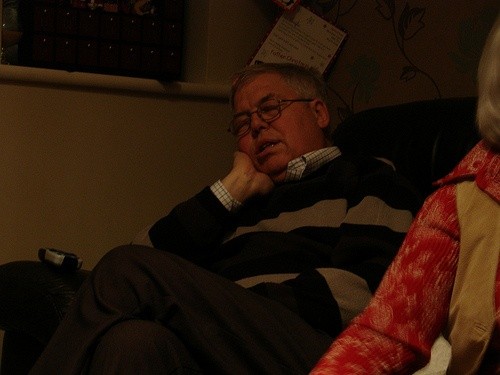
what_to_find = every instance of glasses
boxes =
[227,97,315,137]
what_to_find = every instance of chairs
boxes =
[0,95,481,375]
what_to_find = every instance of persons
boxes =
[15,61,427,375]
[307,11,500,375]
